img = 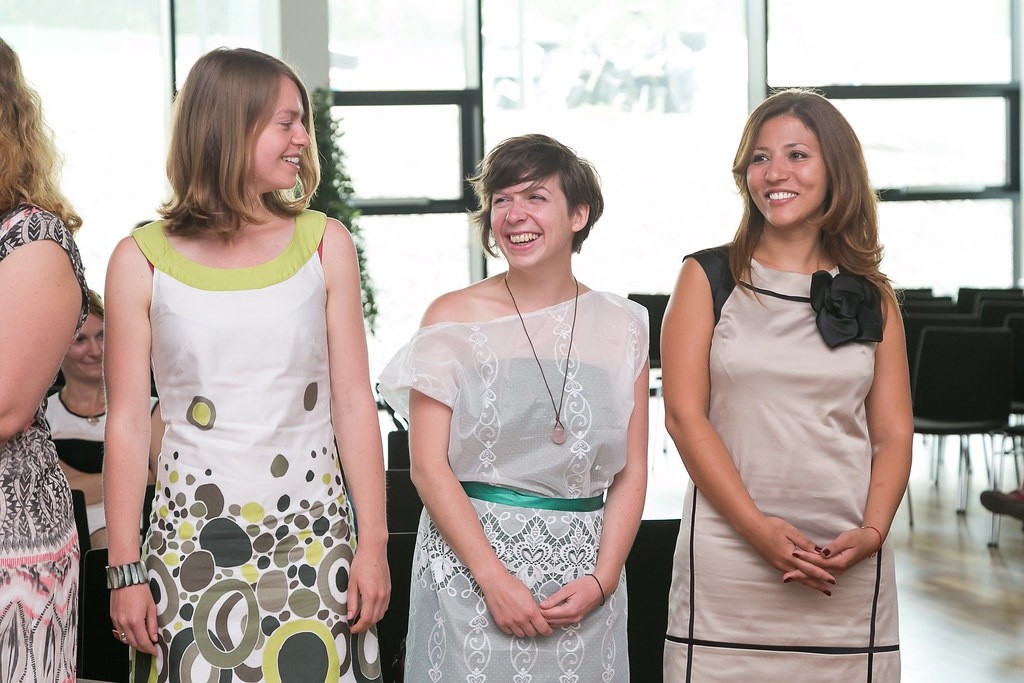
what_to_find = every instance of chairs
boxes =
[627,294,673,452]
[68,486,156,683]
[625,518,681,683]
[892,287,1024,548]
[377,429,423,683]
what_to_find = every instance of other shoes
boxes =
[980,490,1024,521]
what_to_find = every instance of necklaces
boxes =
[87,412,99,425]
[504,274,579,444]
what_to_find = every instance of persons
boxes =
[103,48,391,683]
[376,134,650,683]
[0,40,90,683]
[662,90,914,683]
[46,288,165,549]
[980,480,1024,521]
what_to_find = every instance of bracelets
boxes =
[585,574,605,606]
[105,560,148,589]
[862,525,882,559]
